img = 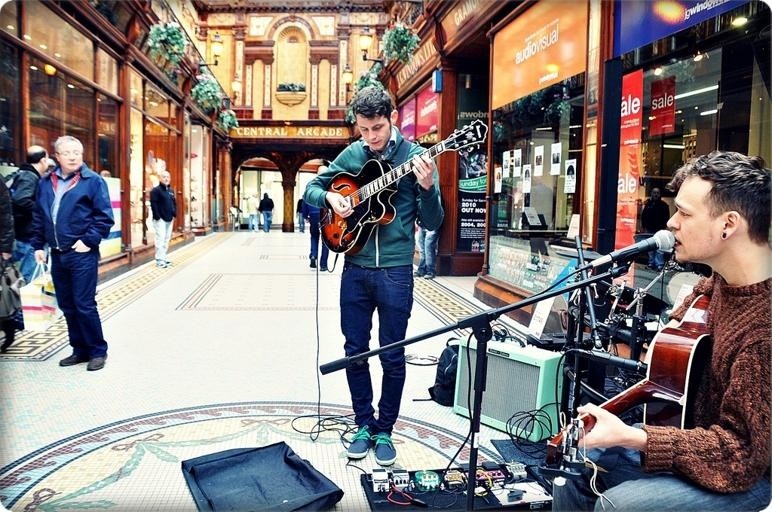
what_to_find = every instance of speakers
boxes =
[453,333,563,443]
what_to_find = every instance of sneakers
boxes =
[347,425,372,459]
[155,260,172,270]
[299,229,304,232]
[14,319,25,331]
[413,270,423,277]
[310,256,316,268]
[320,265,328,271]
[248,229,270,233]
[87,354,108,370]
[59,353,87,367]
[423,272,434,280]
[0,319,17,350]
[648,263,665,271]
[371,432,399,464]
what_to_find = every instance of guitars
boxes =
[551,292,713,446]
[320,119,489,254]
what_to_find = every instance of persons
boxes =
[302,85,445,466]
[0,179,24,356]
[414,226,440,280]
[552,150,772,512]
[304,165,331,271]
[149,171,177,269]
[523,176,556,268]
[10,145,50,286]
[641,188,670,270]
[31,135,115,371]
[296,195,307,233]
[258,193,274,232]
[247,193,260,232]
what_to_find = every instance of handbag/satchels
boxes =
[18,273,64,331]
[0,266,25,317]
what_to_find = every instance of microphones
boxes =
[587,228,675,272]
